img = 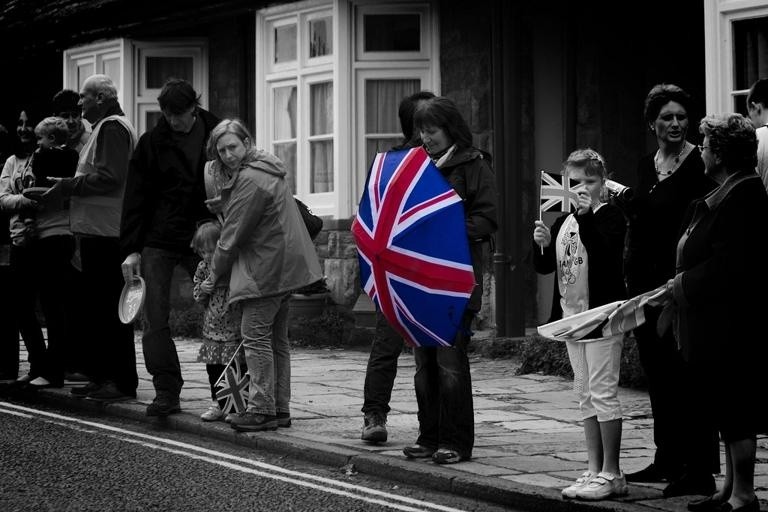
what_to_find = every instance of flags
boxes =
[216,346,248,416]
[536,284,666,344]
[540,169,590,214]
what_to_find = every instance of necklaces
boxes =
[686,203,703,236]
[654,147,687,177]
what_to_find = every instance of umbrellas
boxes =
[349,146,478,351]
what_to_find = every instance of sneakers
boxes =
[70,385,93,399]
[87,384,134,404]
[621,462,680,484]
[147,382,182,415]
[200,401,224,420]
[225,408,293,428]
[432,440,469,464]
[559,469,596,499]
[663,473,717,497]
[575,473,628,502]
[231,411,278,435]
[17,373,29,384]
[30,376,65,389]
[361,411,387,443]
[404,439,437,458]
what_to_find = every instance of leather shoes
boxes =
[687,490,733,511]
[716,496,758,512]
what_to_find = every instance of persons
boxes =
[359,92,439,442]
[401,95,500,462]
[0,74,138,403]
[532,148,630,501]
[188,221,248,425]
[662,112,768,512]
[207,118,325,431]
[624,83,721,498]
[745,77,768,197]
[118,78,224,415]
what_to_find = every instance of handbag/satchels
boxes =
[296,199,324,241]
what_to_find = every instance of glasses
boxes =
[697,144,720,153]
